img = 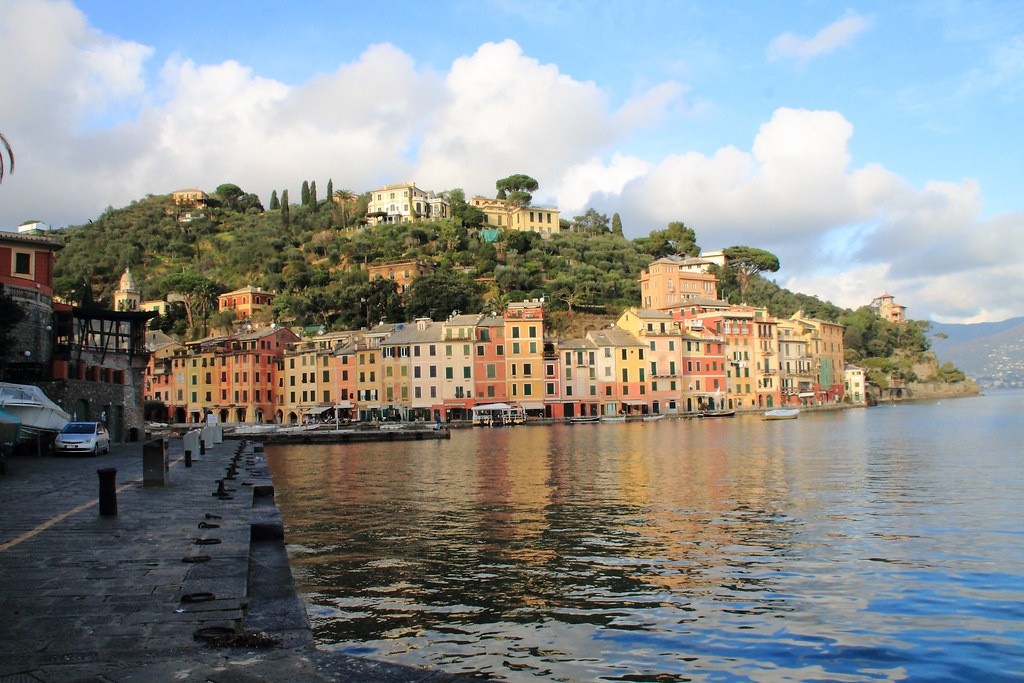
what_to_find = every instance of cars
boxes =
[54,421,112,457]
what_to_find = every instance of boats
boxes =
[697,408,736,418]
[569,415,600,424]
[0,380,72,446]
[761,408,801,421]
